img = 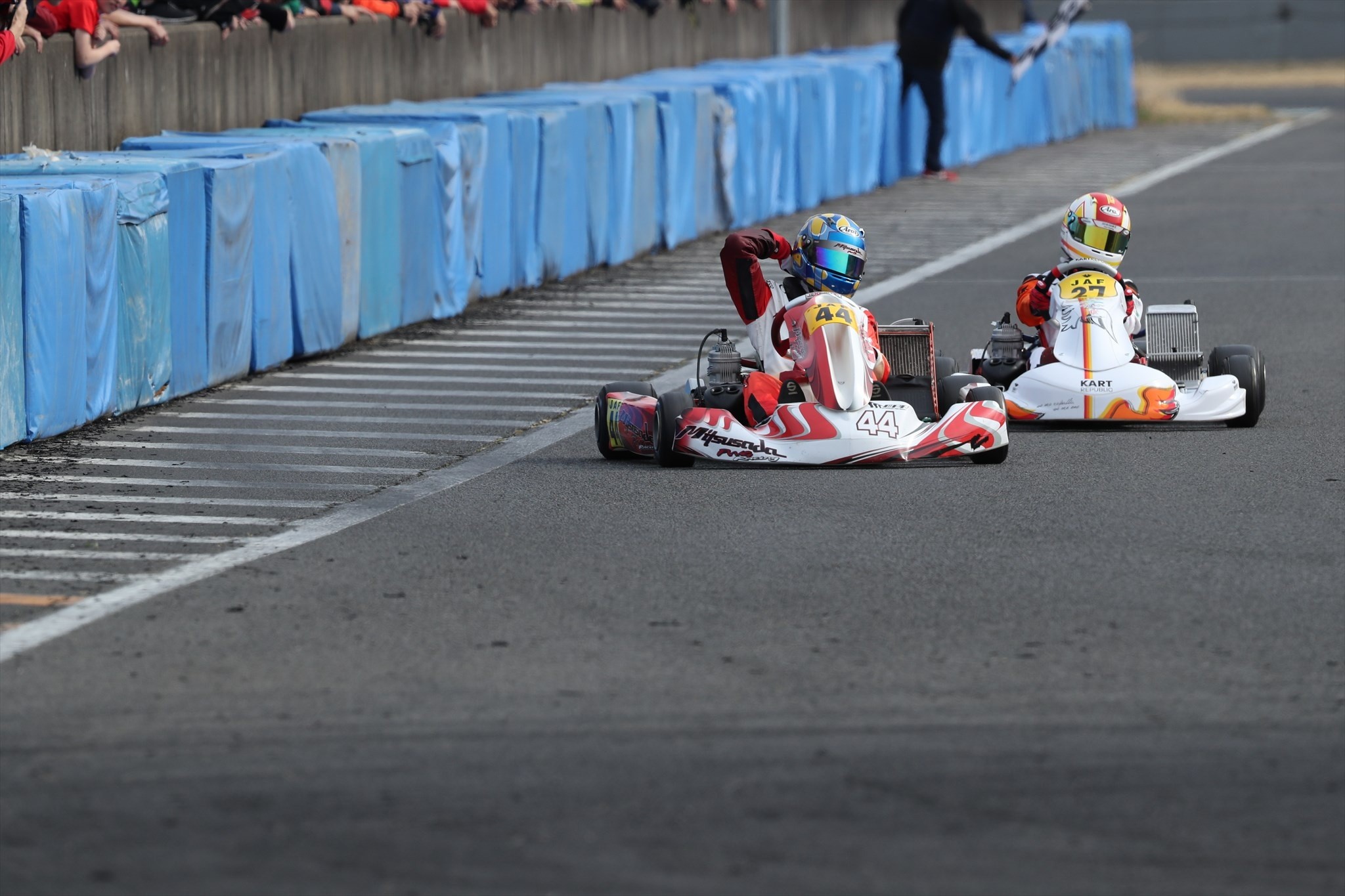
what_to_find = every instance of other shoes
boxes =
[922,170,957,182]
[777,377,806,405]
[1036,347,1058,366]
[871,380,892,401]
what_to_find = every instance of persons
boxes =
[719,213,891,427]
[895,0,1019,180]
[0,0,764,69]
[1016,193,1146,369]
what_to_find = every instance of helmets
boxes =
[1061,192,1130,268]
[793,213,866,299]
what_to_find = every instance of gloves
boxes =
[1029,280,1051,316]
[761,227,793,276]
[1124,287,1134,321]
